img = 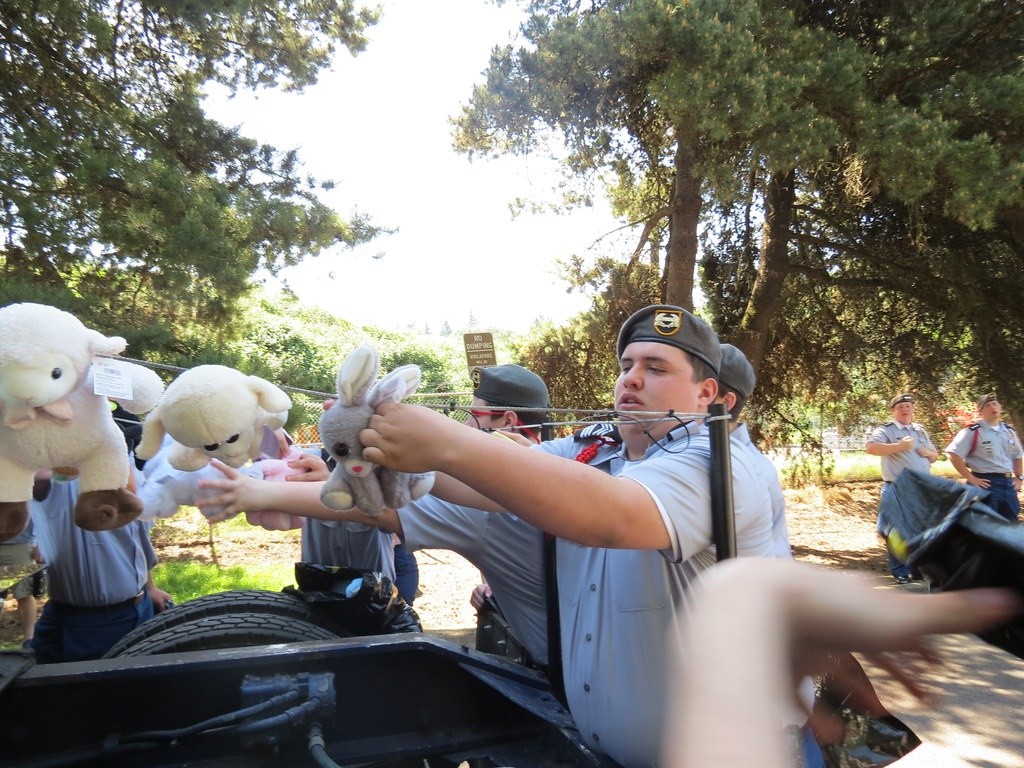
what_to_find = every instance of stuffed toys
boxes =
[0,303,165,540]
[136,364,292,468]
[135,433,307,530]
[319,344,435,515]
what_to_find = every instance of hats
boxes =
[890,394,913,407]
[616,304,721,374]
[470,362,548,408]
[977,392,997,410]
[717,343,756,397]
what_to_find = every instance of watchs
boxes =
[1016,474,1023,481]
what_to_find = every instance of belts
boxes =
[48,590,145,614]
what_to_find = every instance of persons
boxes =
[946,392,1023,523]
[196,363,551,672]
[27,481,172,664]
[866,393,938,583]
[285,305,823,768]
[660,557,1012,768]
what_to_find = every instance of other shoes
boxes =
[895,576,912,584]
[909,574,923,582]
